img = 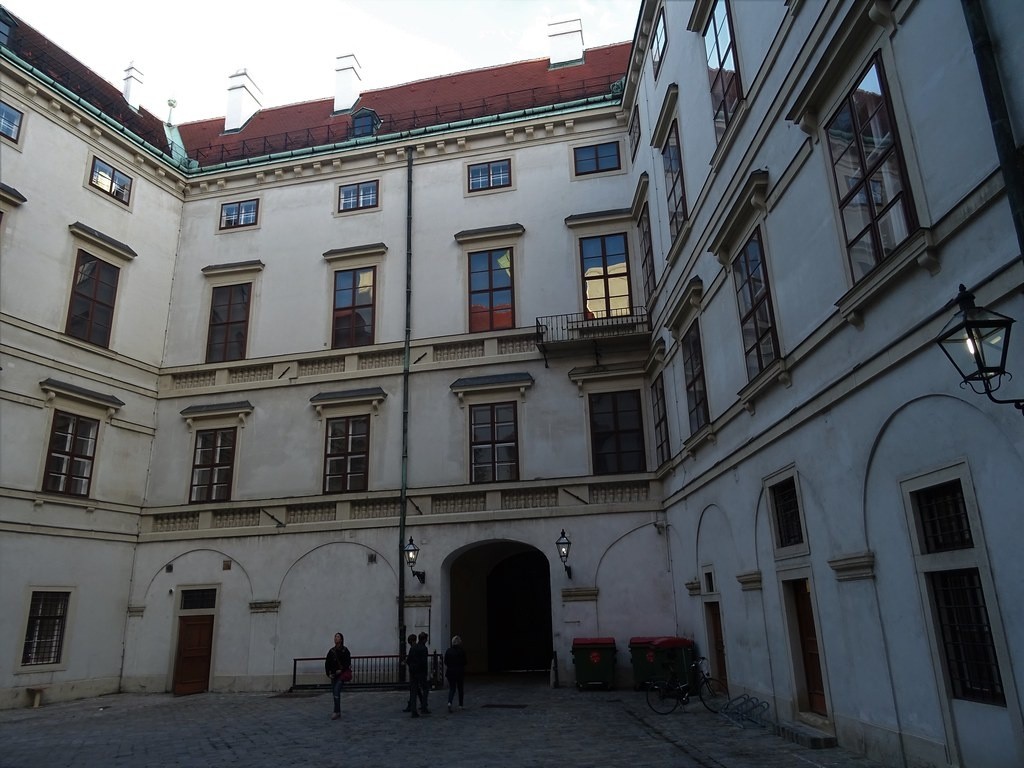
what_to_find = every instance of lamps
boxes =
[934,282,1024,416]
[555,528,574,580]
[402,535,426,584]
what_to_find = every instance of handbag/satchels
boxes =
[340,668,353,682]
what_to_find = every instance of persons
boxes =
[444,636,467,712]
[325,633,351,720]
[401,634,423,712]
[409,632,432,718]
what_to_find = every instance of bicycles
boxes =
[646,656,730,716]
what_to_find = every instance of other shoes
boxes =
[455,705,463,710]
[447,702,453,713]
[411,712,423,718]
[418,705,433,714]
[331,712,341,720]
[402,705,417,713]
